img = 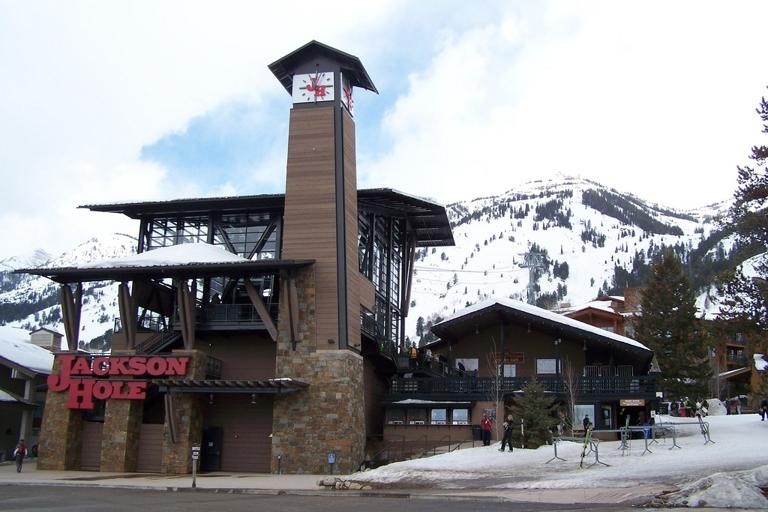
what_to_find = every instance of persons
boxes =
[669,395,744,417]
[498,414,515,453]
[13,437,28,472]
[212,292,221,303]
[379,338,466,384]
[760,397,768,421]
[481,413,493,444]
[582,413,593,439]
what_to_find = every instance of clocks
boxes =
[291,59,336,102]
[340,73,355,118]
